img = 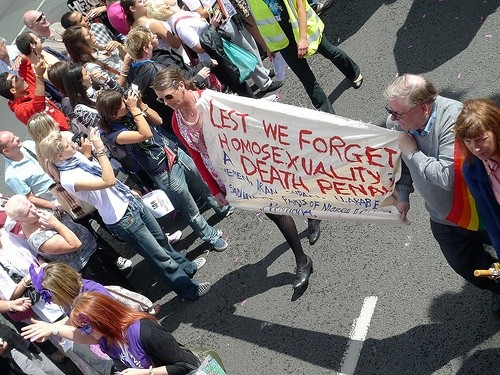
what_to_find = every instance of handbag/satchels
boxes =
[0,262,40,306]
[179,354,227,375]
[47,159,97,220]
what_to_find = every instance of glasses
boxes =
[385,104,420,119]
[31,12,44,26]
[156,85,179,103]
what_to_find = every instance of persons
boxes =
[385,73,500,315]
[0,0,364,375]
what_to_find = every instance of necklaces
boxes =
[177,89,200,127]
[179,100,196,116]
[484,159,500,184]
[39,28,63,41]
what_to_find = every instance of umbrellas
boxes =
[474,263,500,283]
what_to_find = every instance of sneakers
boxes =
[116,256,132,270]
[167,230,182,245]
[177,249,187,257]
[314,0,332,15]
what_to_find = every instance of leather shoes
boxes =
[353,77,363,89]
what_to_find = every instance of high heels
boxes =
[307,219,320,244]
[293,255,314,289]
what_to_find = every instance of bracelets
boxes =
[149,366,153,375]
[52,323,58,336]
[95,149,106,157]
[22,276,31,287]
[122,69,128,73]
[8,300,14,311]
[141,104,148,116]
[120,73,128,77]
[133,112,143,118]
[35,74,43,78]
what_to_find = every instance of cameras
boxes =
[124,85,133,99]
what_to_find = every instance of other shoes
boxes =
[213,238,228,251]
[264,80,283,93]
[192,256,207,270]
[223,205,234,217]
[197,283,210,297]
[491,292,500,314]
[268,68,275,78]
